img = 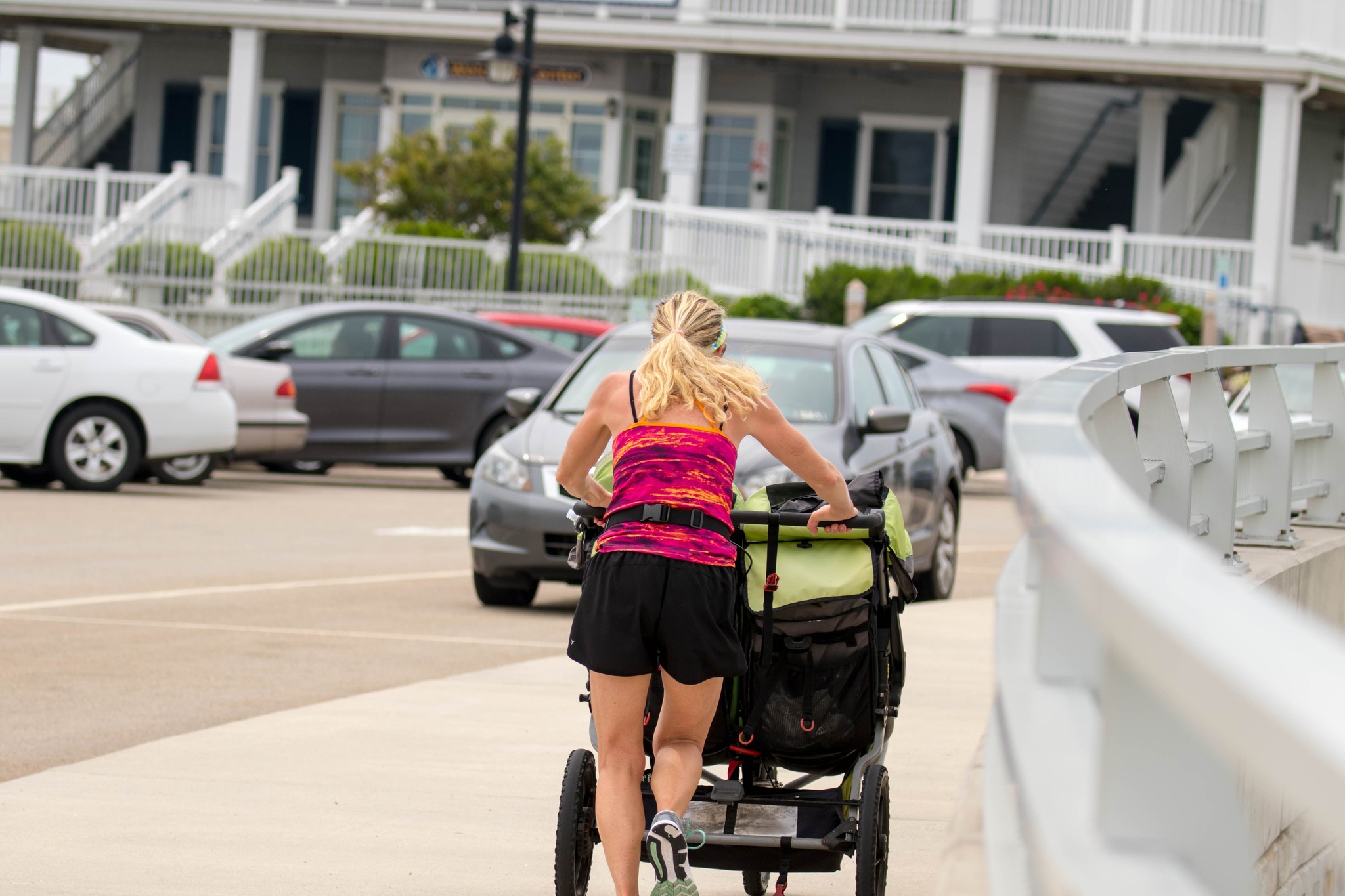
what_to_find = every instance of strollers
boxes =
[554,449,921,896]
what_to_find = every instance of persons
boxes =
[556,290,859,896]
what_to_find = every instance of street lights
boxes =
[482,1,547,289]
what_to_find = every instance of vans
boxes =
[857,298,1195,440]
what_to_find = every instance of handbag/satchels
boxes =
[582,452,911,776]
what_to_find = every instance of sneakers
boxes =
[645,809,699,896]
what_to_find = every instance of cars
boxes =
[73,298,309,486]
[469,317,961,610]
[873,336,1018,483]
[0,285,243,491]
[399,311,619,482]
[211,299,579,488]
